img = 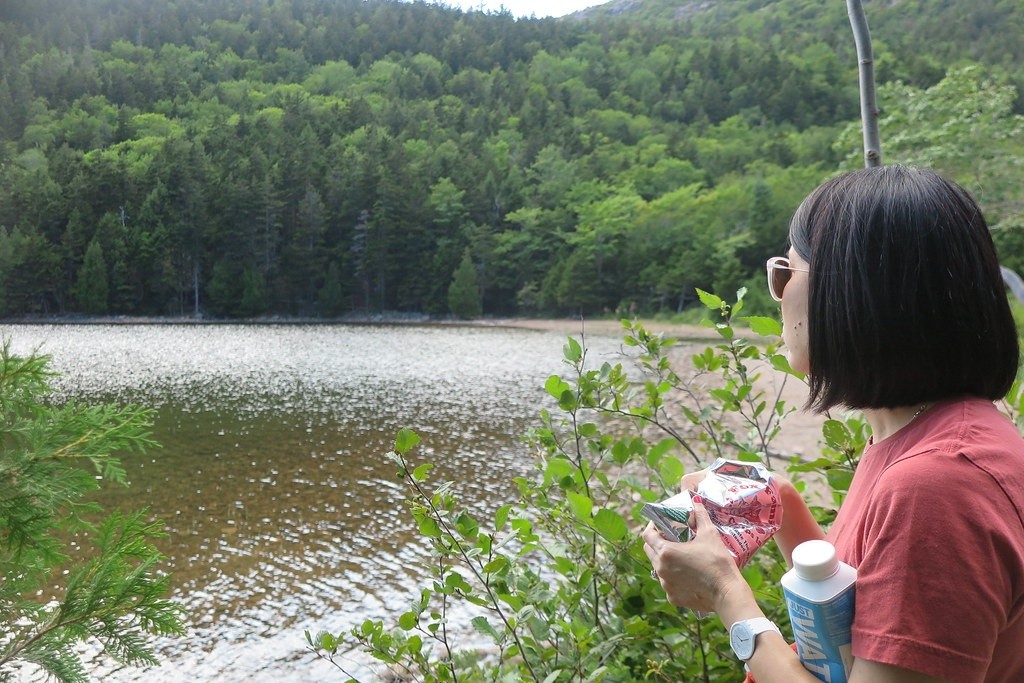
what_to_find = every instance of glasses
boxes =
[766,257,810,302]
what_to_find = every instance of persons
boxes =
[642,167,1024,683]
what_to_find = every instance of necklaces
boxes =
[913,402,928,418]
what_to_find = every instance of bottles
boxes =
[780,539,857,683]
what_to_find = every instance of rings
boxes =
[651,569,658,579]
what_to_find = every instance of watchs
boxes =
[730,618,783,672]
